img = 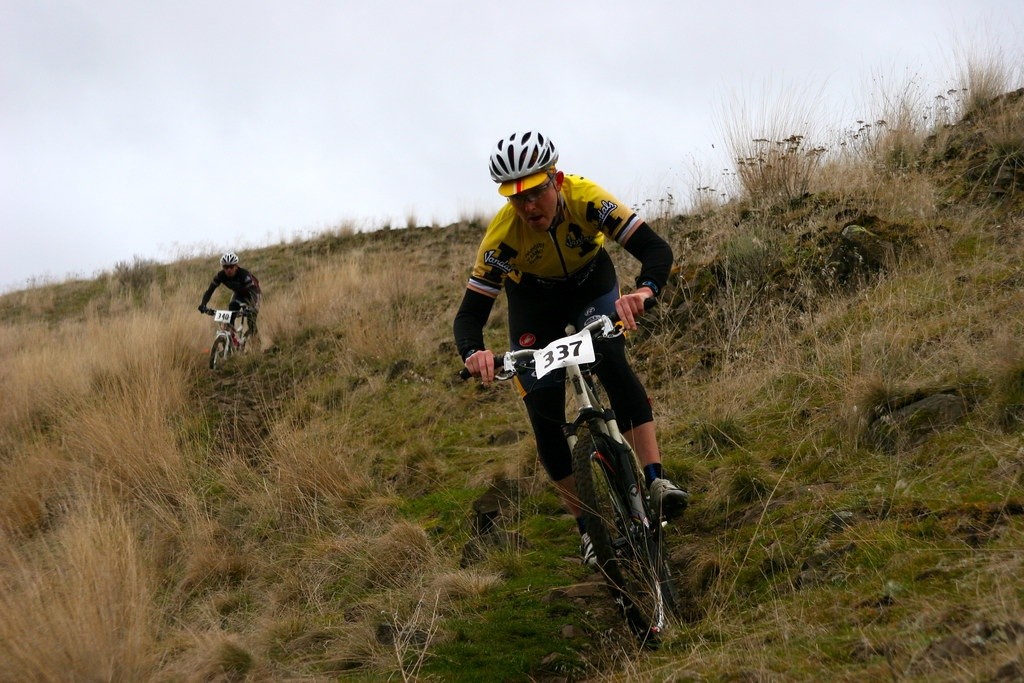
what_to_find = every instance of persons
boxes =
[198,252,261,342]
[453,128,689,567]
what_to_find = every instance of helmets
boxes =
[490,129,558,183]
[220,253,238,265]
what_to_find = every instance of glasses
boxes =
[221,264,236,269]
[507,173,555,204]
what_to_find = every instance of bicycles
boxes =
[198,303,262,370]
[459,298,683,648]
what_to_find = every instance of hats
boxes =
[498,165,556,198]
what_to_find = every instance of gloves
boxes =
[199,303,207,315]
[243,309,251,316]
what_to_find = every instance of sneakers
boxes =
[649,478,688,519]
[580,531,603,568]
